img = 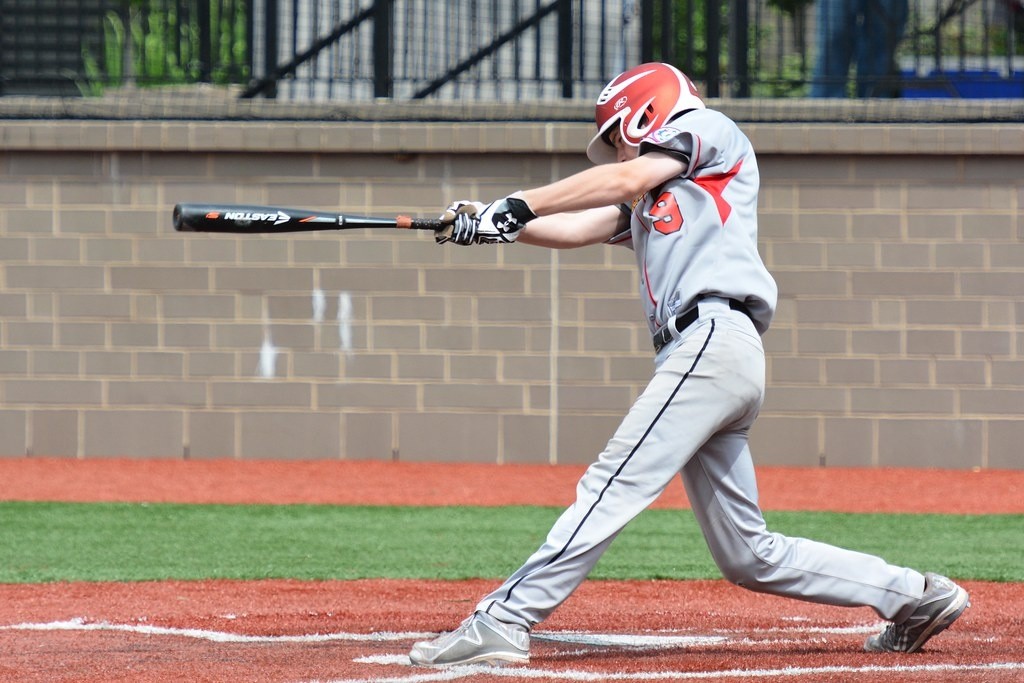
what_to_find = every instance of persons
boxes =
[811,0,912,100]
[409,62,969,670]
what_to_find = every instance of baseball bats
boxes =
[173,202,449,234]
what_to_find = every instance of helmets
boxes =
[585,62,707,166]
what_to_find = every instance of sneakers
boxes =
[409,609,530,669]
[863,572,969,654]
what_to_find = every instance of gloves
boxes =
[434,190,537,247]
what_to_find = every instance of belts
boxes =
[652,296,752,355]
